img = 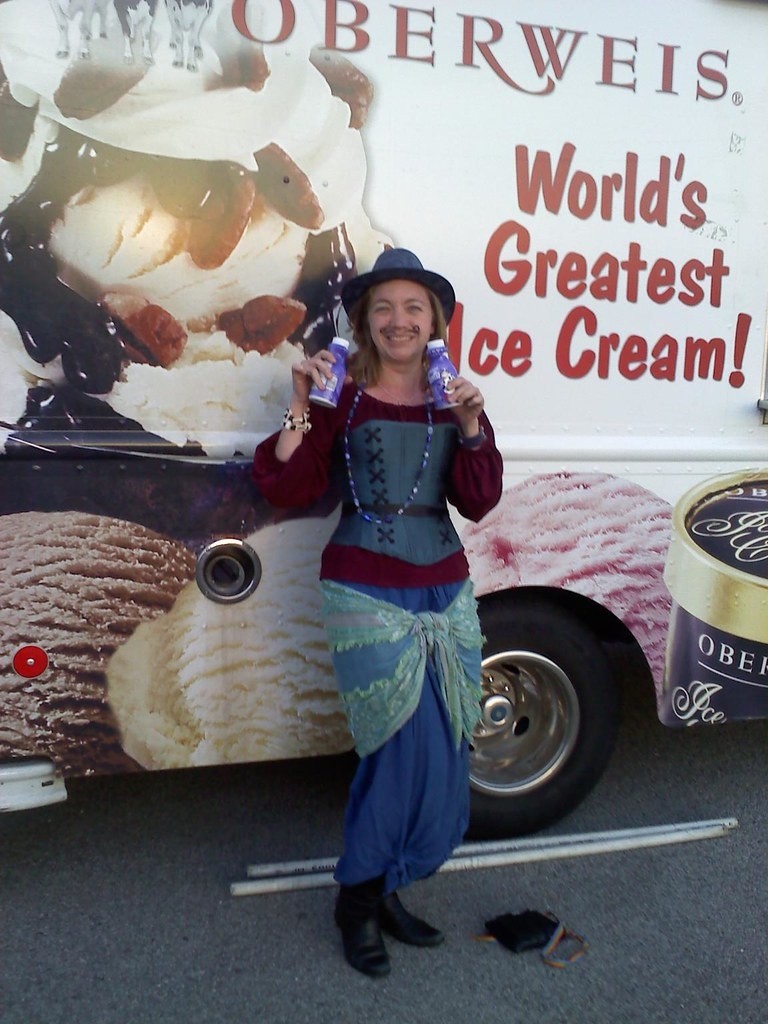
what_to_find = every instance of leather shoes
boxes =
[379,891,444,947]
[334,881,391,978]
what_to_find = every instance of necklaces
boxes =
[344,375,434,524]
[372,367,426,404]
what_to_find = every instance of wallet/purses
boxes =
[486,911,559,954]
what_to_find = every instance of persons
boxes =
[249,249,503,978]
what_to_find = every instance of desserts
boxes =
[106,519,353,771]
[0,0,391,447]
[459,474,675,698]
[0,512,200,758]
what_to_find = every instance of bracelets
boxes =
[457,424,487,447]
[281,409,312,434]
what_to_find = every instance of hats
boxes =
[341,249,456,327]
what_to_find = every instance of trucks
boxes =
[1,0,768,843]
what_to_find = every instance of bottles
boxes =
[309,338,349,407]
[426,338,462,409]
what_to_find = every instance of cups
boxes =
[661,470,768,725]
